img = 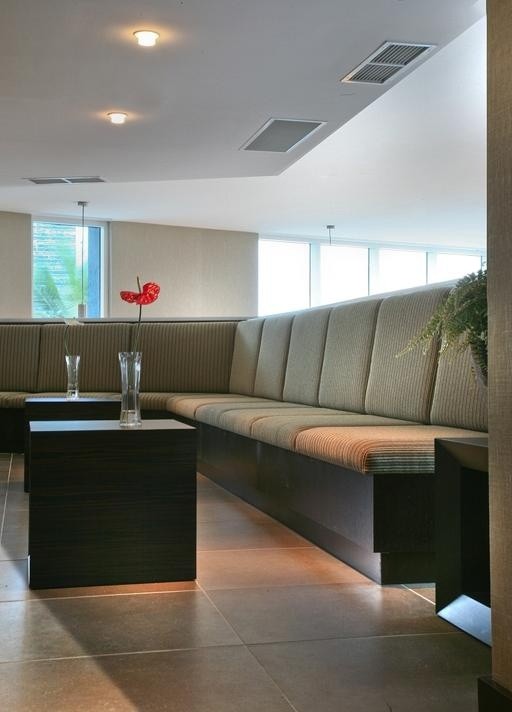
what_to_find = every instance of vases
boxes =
[117,351,143,428]
[65,355,81,399]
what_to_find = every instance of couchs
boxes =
[0,279,486,586]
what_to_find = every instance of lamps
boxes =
[133,30,158,49]
[107,112,128,124]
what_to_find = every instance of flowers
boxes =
[63,317,85,357]
[120,275,160,350]
